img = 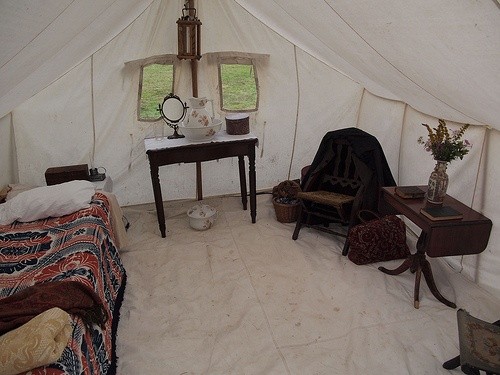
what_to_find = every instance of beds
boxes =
[0,193,128,375]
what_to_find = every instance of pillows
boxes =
[0,180,95,225]
[0,307,74,375]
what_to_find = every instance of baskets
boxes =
[273,180,302,223]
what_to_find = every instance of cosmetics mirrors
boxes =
[156,93,189,138]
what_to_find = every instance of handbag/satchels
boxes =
[348,210,406,265]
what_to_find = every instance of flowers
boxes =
[418,119,473,163]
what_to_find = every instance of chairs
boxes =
[443,308,500,375]
[292,127,381,256]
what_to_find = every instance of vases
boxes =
[424,160,449,204]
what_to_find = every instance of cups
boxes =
[153,121,163,141]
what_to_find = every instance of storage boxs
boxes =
[45,164,90,186]
[225,113,250,135]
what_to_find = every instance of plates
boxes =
[187,204,216,218]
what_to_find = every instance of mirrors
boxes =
[156,93,189,139]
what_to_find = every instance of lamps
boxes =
[176,1,202,61]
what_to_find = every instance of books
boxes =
[394,186,426,199]
[420,206,463,221]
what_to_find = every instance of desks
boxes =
[379,185,493,310]
[144,130,259,238]
[5,176,113,193]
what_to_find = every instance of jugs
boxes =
[187,96,215,127]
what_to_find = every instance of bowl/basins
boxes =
[178,118,223,143]
[190,215,214,229]
[225,112,250,135]
